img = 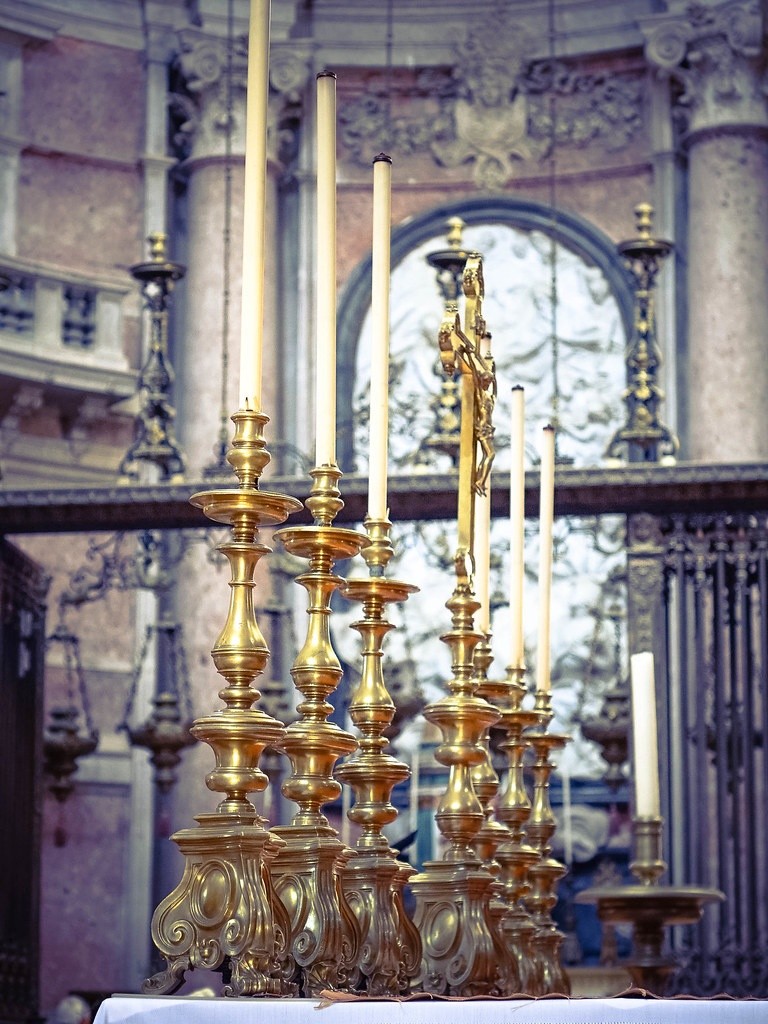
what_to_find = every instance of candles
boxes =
[629,649,659,818]
